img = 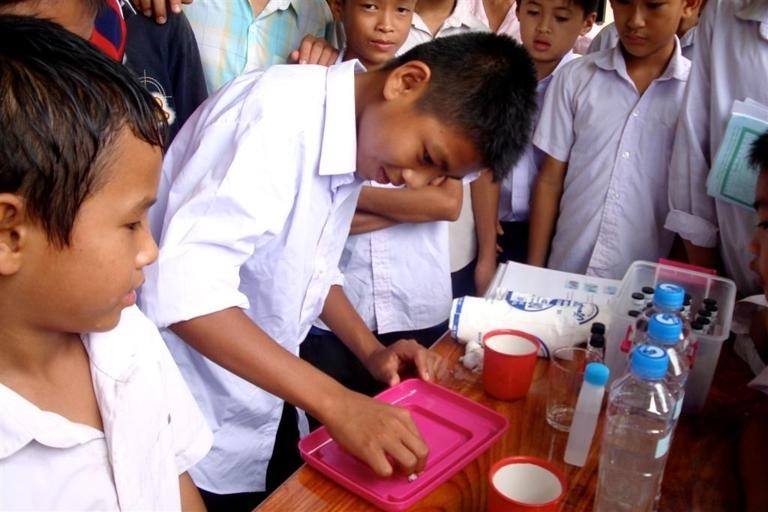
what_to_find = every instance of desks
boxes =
[242,326,742,510]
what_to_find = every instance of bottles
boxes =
[564,278,719,512]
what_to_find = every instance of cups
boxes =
[481,328,541,402]
[545,346,605,432]
[485,452,569,512]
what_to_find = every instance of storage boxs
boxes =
[604,261,738,413]
